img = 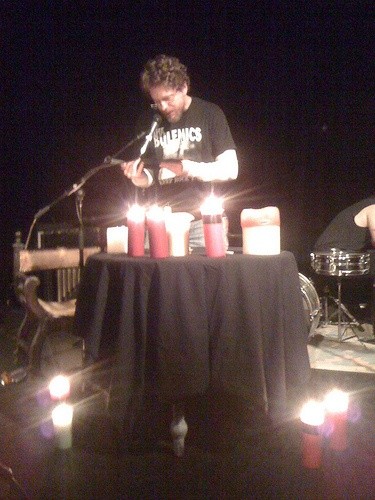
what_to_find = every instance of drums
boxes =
[309,248,371,276]
[297,273,321,343]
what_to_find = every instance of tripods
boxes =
[316,282,368,349]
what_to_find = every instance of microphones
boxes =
[140,112,162,155]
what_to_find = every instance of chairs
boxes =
[14,272,76,374]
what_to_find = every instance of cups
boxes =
[240,206,281,256]
[168,212,192,257]
[125,216,146,257]
[107,226,128,255]
[148,217,167,258]
[202,213,225,258]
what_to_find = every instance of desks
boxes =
[71,250,311,448]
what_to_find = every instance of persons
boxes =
[307,196,375,342]
[119,54,239,249]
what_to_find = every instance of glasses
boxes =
[150,92,177,110]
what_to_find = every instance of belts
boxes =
[191,212,203,222]
